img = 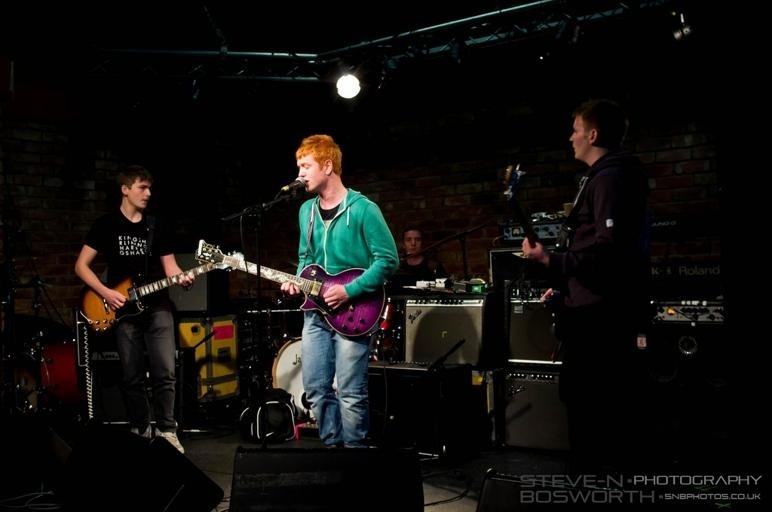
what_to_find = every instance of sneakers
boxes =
[130,423,153,440]
[154,425,187,455]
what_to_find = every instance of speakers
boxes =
[227,447,424,512]
[45,419,224,512]
[508,300,565,369]
[505,368,570,450]
[475,474,720,512]
[405,299,484,366]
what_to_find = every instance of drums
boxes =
[378,296,396,331]
[271,336,338,429]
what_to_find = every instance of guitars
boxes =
[194,239,386,337]
[79,250,245,336]
[502,162,561,336]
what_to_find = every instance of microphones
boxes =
[279,176,306,191]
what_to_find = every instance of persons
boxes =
[279,131,399,444]
[74,166,196,456]
[522,99,652,478]
[391,227,453,292]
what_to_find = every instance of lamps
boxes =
[669,12,690,40]
